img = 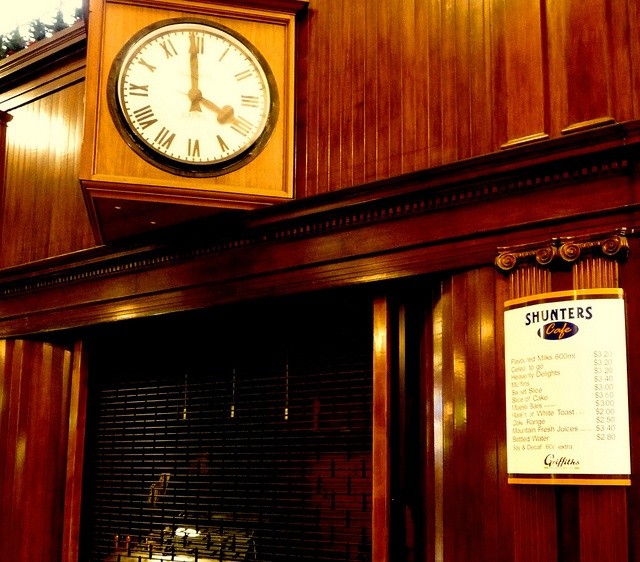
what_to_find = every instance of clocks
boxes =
[105,15,281,179]
